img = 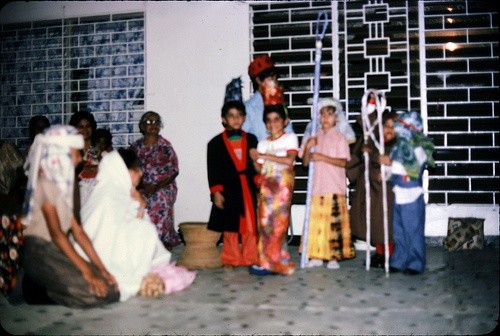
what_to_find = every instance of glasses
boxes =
[142,119,161,125]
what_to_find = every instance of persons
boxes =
[352,112,429,276]
[0,110,197,309]
[299,96,357,270]
[207,59,298,275]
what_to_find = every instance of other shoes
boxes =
[306,259,323,267]
[389,267,400,273]
[327,261,340,269]
[365,253,391,268]
[403,268,419,275]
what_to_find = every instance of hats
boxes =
[248,53,274,80]
